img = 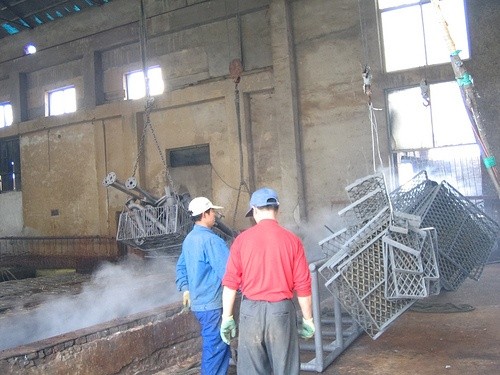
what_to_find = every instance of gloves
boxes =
[220,314,236,345]
[302,317,316,338]
[182,291,190,314]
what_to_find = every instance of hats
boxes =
[188,197,224,217]
[245,187,280,217]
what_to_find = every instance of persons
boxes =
[220,188,315,375]
[175,197,232,375]
